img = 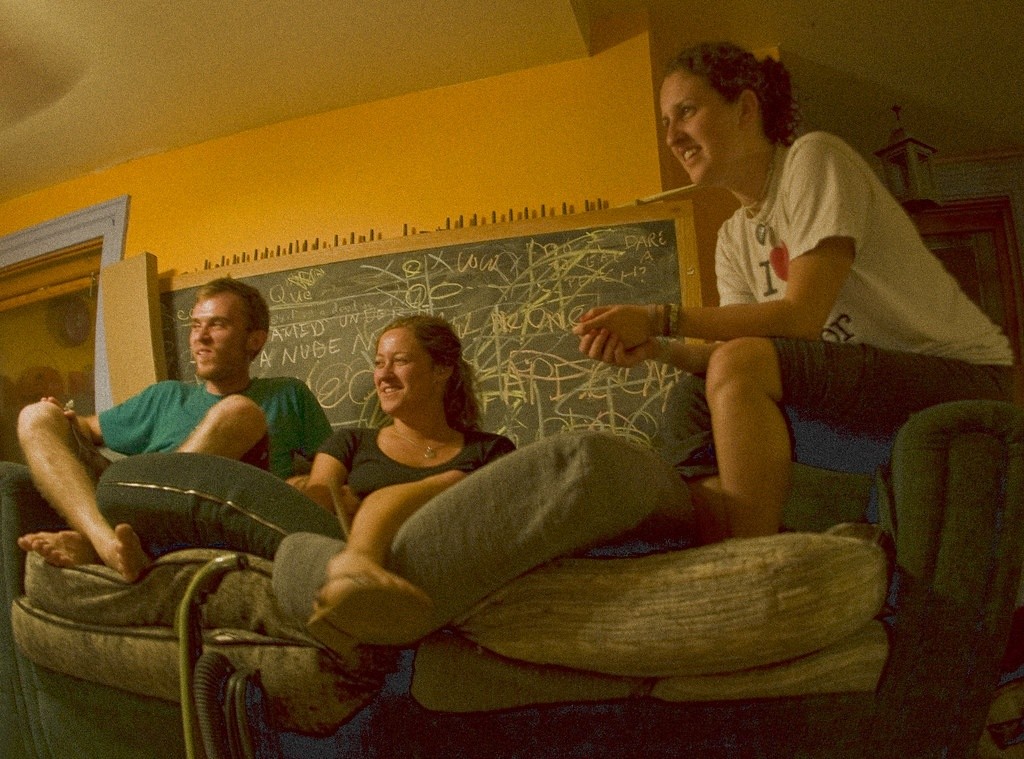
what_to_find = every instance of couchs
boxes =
[0,399,1024,759]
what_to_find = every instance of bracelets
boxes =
[647,302,656,336]
[664,303,685,338]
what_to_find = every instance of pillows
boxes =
[272,429,697,649]
[95,451,347,562]
[782,455,879,536]
[448,520,899,680]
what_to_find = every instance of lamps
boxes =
[872,104,944,213]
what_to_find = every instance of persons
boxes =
[16,278,334,586]
[570,41,1014,539]
[301,312,517,661]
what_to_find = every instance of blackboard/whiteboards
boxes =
[156,199,716,456]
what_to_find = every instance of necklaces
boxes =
[393,427,447,459]
[743,163,773,244]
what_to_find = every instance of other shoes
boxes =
[308,548,434,656]
